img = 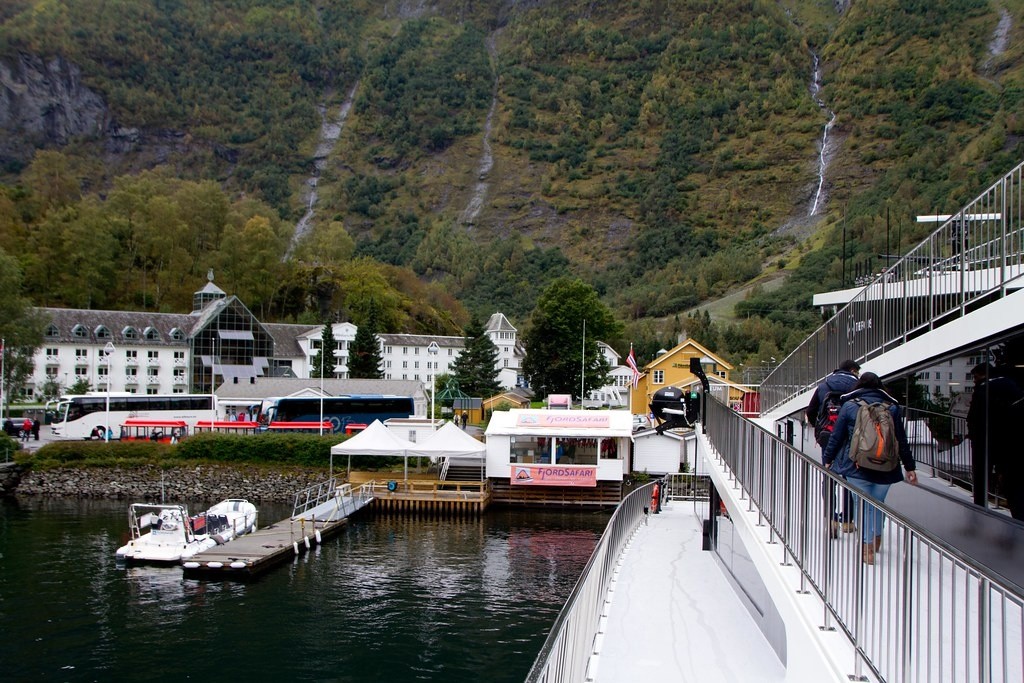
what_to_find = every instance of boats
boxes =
[115,499,257,567]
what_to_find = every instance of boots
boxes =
[876,536,880,551]
[863,543,874,565]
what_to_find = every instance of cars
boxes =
[633,414,652,434]
[2,418,34,438]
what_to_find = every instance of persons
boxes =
[453,412,461,427]
[806,359,862,541]
[963,364,1024,521]
[93,426,178,442]
[823,372,918,566]
[461,410,469,429]
[556,440,563,464]
[3,416,41,442]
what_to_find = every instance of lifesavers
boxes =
[388,481,396,491]
[651,483,658,513]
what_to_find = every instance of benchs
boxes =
[334,483,352,496]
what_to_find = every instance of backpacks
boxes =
[814,382,844,449]
[847,397,899,472]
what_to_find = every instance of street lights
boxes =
[427,341,441,434]
[104,342,117,443]
[739,356,789,384]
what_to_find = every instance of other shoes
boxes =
[830,520,837,538]
[844,522,855,532]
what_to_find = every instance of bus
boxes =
[45,392,219,440]
[249,394,414,434]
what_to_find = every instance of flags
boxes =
[626,347,639,389]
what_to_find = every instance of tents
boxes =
[329,419,418,492]
[405,421,487,480]
